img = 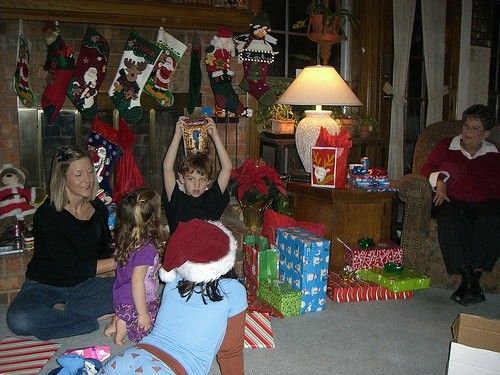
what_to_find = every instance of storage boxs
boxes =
[274,228,330,312]
[358,261,431,293]
[446,313,500,375]
[259,283,301,317]
[326,269,412,302]
[345,237,403,273]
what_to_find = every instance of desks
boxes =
[260,136,388,177]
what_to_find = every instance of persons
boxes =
[95,218,248,375]
[103,187,163,345]
[6,145,117,341]
[162,115,233,238]
[419,104,500,306]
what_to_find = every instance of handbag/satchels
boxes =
[244,233,281,295]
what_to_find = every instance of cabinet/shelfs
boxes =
[286,182,404,271]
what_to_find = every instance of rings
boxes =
[439,196,442,198]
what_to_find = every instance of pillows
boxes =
[263,208,328,243]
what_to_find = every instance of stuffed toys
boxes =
[233,9,276,54]
[0,163,38,233]
[204,29,235,69]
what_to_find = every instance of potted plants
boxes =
[335,108,370,138]
[303,0,361,43]
[257,100,297,134]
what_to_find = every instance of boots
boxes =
[450,266,486,306]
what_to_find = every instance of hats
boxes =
[159,217,239,284]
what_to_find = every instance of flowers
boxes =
[227,158,287,206]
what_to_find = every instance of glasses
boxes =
[459,121,486,134]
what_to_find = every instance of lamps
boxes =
[275,64,363,174]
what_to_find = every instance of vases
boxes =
[240,200,268,228]
